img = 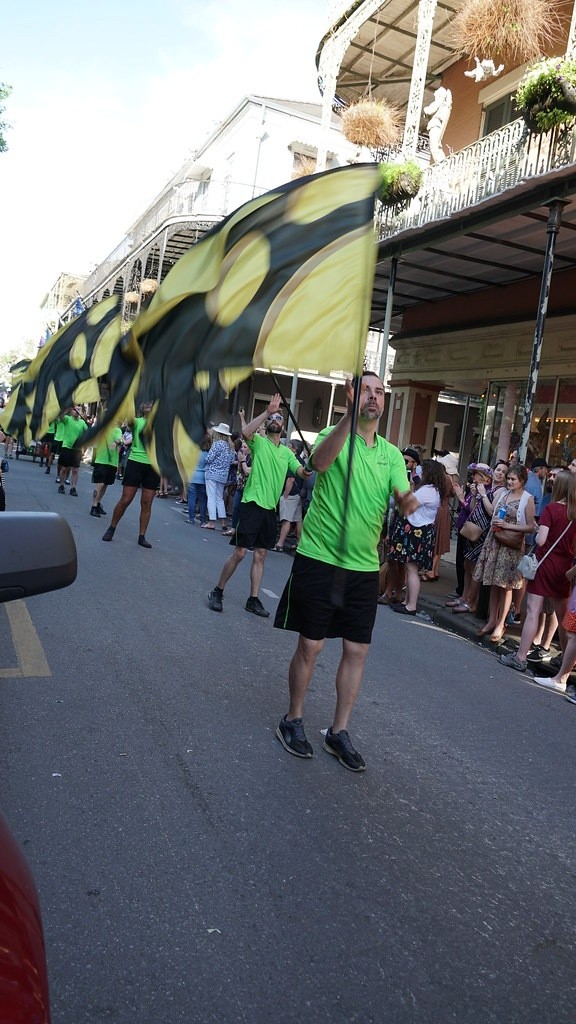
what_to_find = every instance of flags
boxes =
[0,163,382,490]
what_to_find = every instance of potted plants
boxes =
[374,163,422,204]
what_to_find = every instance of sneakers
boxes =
[275,713,313,757]
[208,589,223,611]
[245,598,270,616]
[323,725,367,771]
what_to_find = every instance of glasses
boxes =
[546,473,556,478]
[497,459,511,467]
[404,459,415,464]
[267,416,283,421]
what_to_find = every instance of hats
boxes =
[402,449,420,466]
[531,459,552,469]
[467,462,493,478]
[212,423,232,435]
[436,454,460,476]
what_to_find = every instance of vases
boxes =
[523,96,565,133]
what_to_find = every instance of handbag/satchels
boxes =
[460,521,481,542]
[516,555,538,580]
[495,527,524,549]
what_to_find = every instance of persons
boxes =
[270,429,315,552]
[102,401,162,549]
[89,428,122,518]
[272,369,419,771]
[378,445,576,705]
[39,421,55,467]
[5,434,14,459]
[44,417,65,474]
[423,87,445,162]
[58,403,87,496]
[55,463,70,485]
[81,414,253,535]
[207,393,313,618]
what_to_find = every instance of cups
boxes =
[492,516,499,527]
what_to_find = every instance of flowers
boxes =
[512,52,576,132]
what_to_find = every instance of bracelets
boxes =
[303,465,312,477]
[114,441,118,444]
[241,461,245,463]
[482,495,487,498]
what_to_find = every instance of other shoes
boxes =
[97,504,106,514]
[445,592,576,704]
[39,463,70,485]
[89,511,101,519]
[378,571,440,615]
[58,488,65,493]
[138,538,151,548]
[70,489,77,496]
[155,490,300,552]
[102,529,115,541]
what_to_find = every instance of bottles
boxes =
[496,504,507,521]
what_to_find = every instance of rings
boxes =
[479,484,481,486]
